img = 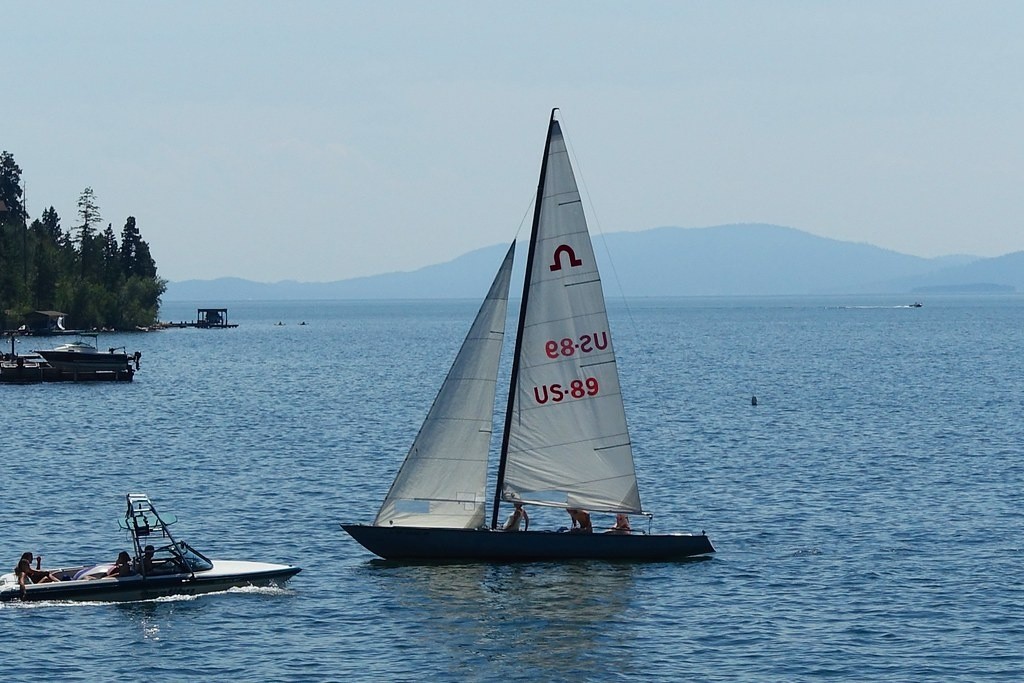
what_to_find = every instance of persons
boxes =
[564,508,632,535]
[502,502,529,532]
[13,551,62,594]
[103,545,173,578]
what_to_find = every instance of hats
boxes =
[118,551,132,561]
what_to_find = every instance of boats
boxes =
[0,492,304,602]
[0,332,142,385]
[909,301,923,308]
[297,320,310,326]
[273,320,286,326]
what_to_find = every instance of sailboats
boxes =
[339,105,717,564]
